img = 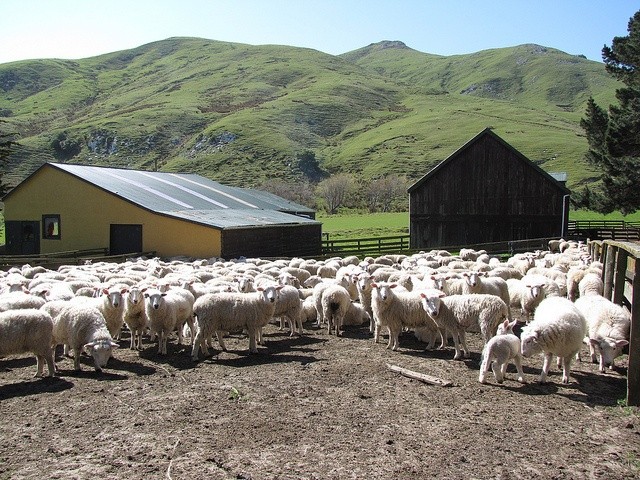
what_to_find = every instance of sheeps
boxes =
[1,238,607,290]
[462,271,512,321]
[352,271,411,336]
[1,289,65,309]
[52,302,120,378]
[520,295,587,385]
[369,280,446,350]
[122,283,154,352]
[253,278,305,345]
[191,283,284,362]
[478,317,527,385]
[573,291,630,373]
[63,288,125,357]
[321,284,351,336]
[0,308,56,378]
[518,280,546,326]
[420,289,509,361]
[144,285,197,356]
